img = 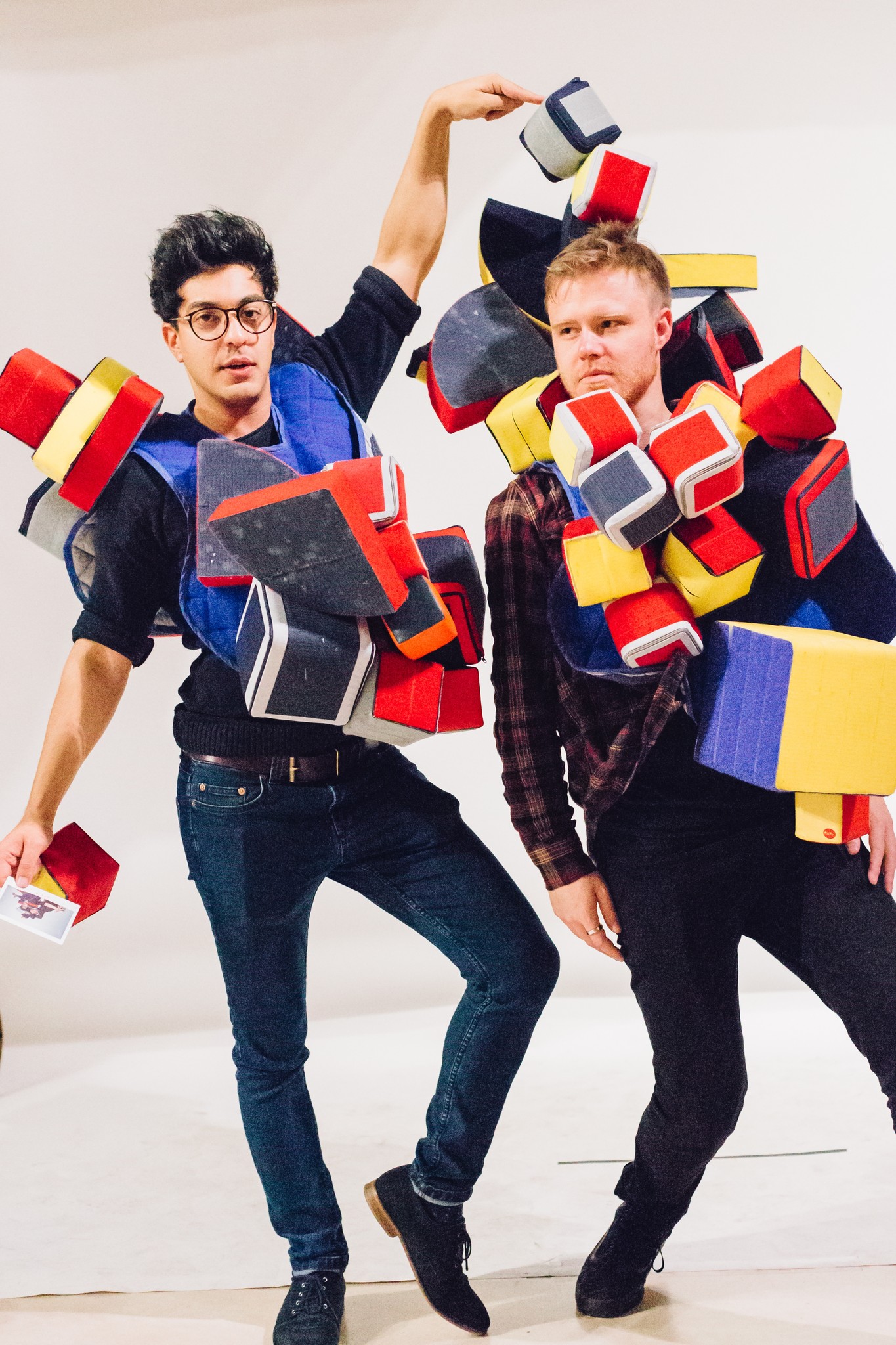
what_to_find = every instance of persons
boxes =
[0,72,560,1345]
[485,220,896,1320]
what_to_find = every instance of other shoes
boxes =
[364,1164,493,1337]
[273,1267,344,1345]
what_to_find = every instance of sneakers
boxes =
[575,1200,683,1318]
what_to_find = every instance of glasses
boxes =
[169,298,278,341]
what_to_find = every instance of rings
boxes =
[586,924,603,936]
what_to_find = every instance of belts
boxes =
[188,736,381,785]
